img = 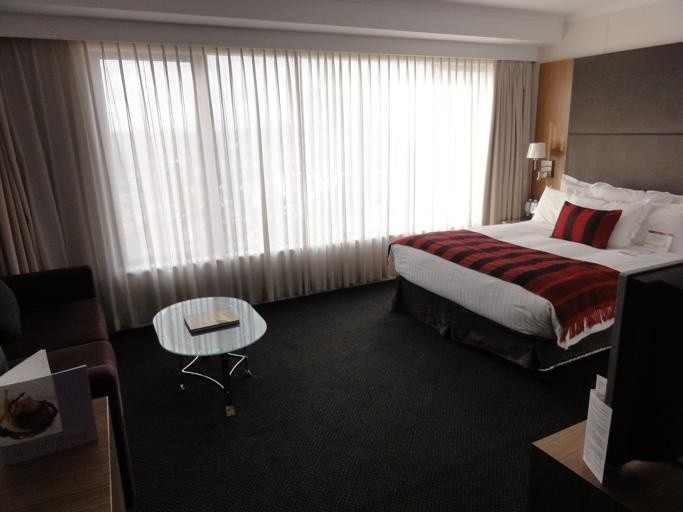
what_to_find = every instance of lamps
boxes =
[525,142,556,179]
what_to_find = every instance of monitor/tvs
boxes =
[604,259,683,473]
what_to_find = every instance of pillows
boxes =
[529,174,682,250]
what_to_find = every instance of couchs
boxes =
[1,260,140,511]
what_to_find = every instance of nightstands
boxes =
[500,217,530,223]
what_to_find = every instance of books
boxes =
[179,305,243,336]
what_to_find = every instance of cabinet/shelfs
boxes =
[525,421,683,512]
[0,392,125,512]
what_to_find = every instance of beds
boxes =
[386,179,682,372]
